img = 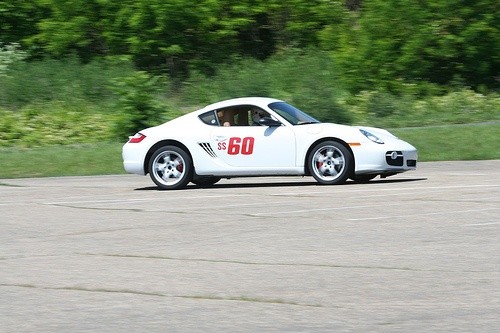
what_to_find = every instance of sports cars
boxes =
[122,97,417,189]
